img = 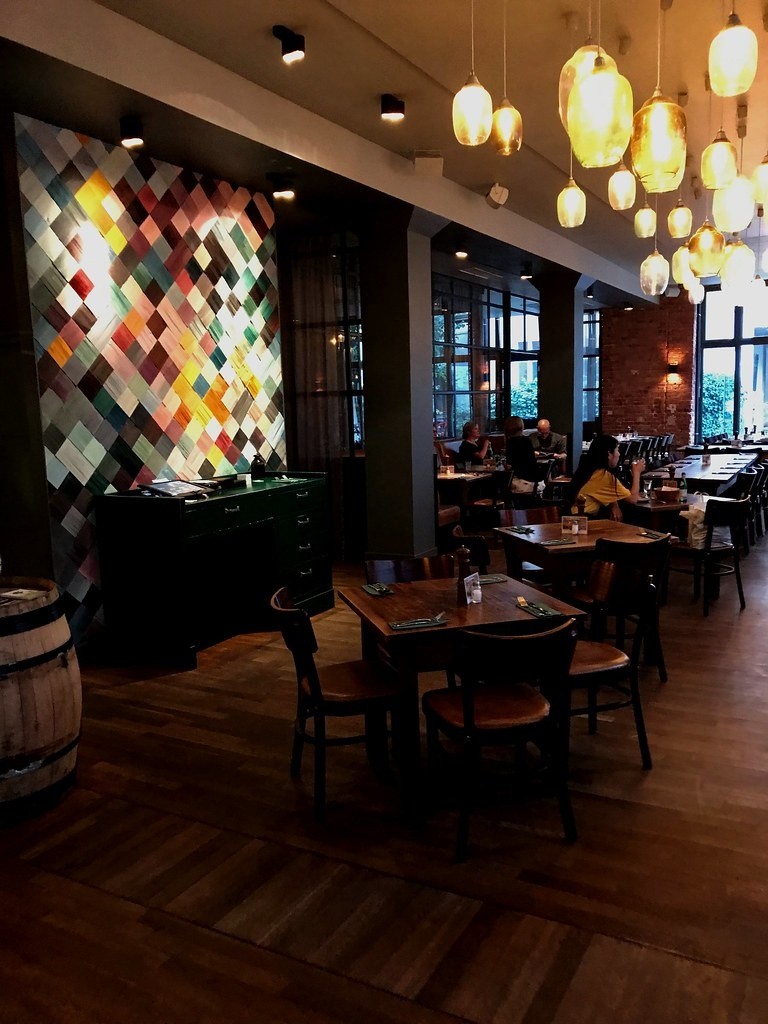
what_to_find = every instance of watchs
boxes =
[558,455,563,461]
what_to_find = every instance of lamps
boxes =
[120,0,768,375]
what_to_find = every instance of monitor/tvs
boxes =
[137,479,215,496]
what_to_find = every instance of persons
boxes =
[460,422,491,465]
[433,423,456,476]
[571,434,647,523]
[504,416,554,500]
[531,419,568,477]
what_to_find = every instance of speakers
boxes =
[665,287,680,297]
[413,156,444,177]
[485,186,509,209]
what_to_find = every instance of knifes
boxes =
[645,529,662,537]
[369,584,383,594]
[525,598,551,614]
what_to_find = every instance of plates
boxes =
[637,498,650,501]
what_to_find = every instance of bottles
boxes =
[679,473,688,502]
[252,455,266,479]
[457,545,471,586]
[663,465,678,487]
[572,521,579,535]
[701,443,710,466]
[487,442,493,457]
[550,456,572,480]
[471,582,482,602]
[744,427,749,440]
[731,430,742,448]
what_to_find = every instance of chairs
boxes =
[235,415,768,865]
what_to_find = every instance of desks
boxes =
[628,492,727,604]
[532,450,556,485]
[437,470,493,520]
[469,461,511,508]
[637,450,759,499]
[336,572,590,822]
[492,516,669,682]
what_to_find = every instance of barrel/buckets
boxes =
[0,575,83,802]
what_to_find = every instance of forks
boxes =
[394,611,446,626]
[505,527,530,534]
[639,528,659,539]
[376,583,390,592]
[517,596,544,617]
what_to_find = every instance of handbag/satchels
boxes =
[679,491,732,549]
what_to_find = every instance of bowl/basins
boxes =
[654,487,679,501]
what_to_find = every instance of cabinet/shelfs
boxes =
[97,471,332,666]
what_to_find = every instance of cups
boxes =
[643,480,652,499]
[632,458,641,476]
